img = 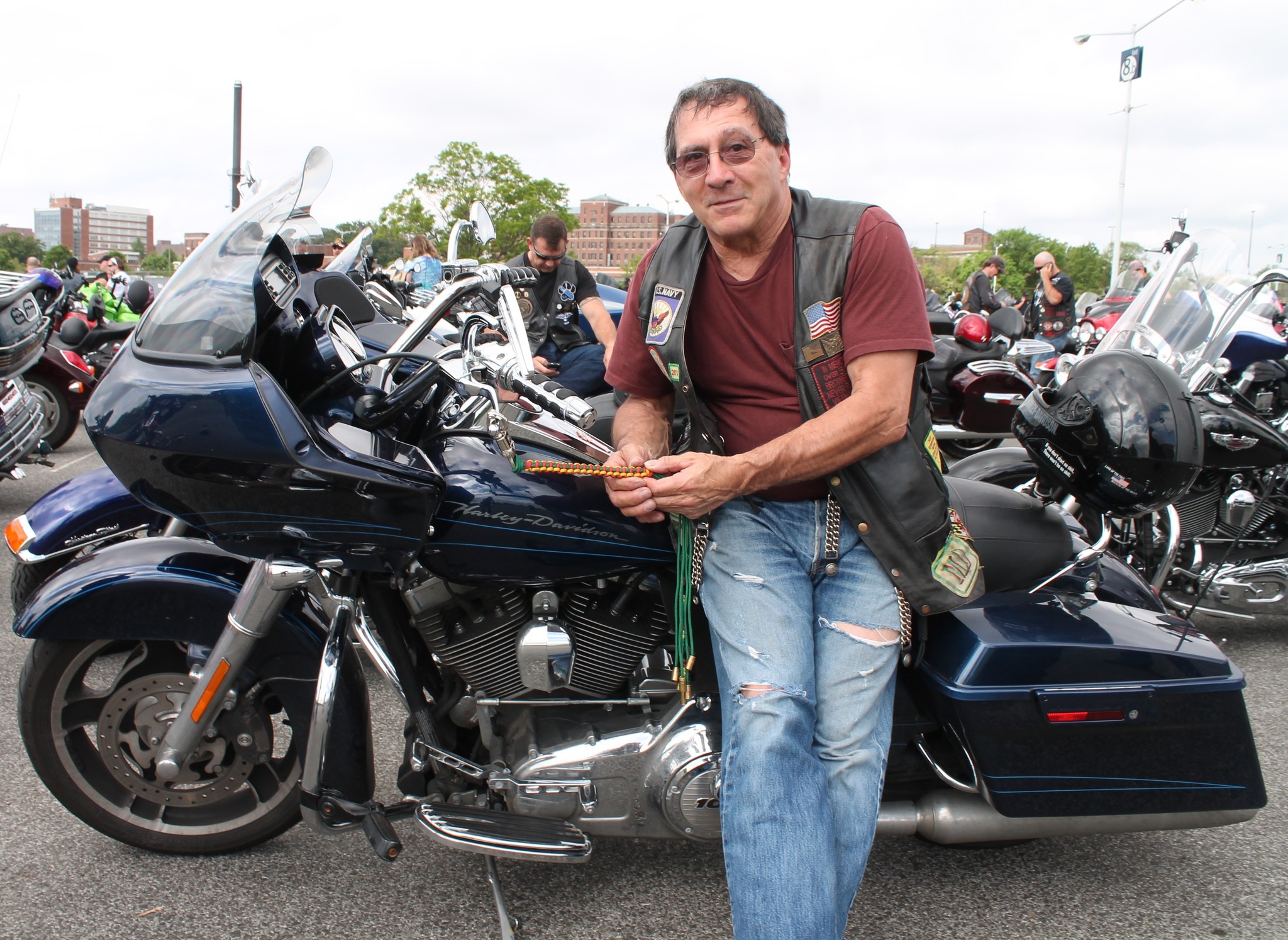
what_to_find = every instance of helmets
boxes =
[953,313,992,351]
[126,280,155,315]
[1011,350,1205,519]
[59,316,89,344]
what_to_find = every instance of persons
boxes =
[321,238,357,271]
[1014,292,1031,315]
[24,255,155,313]
[501,216,618,399]
[1129,260,1150,296]
[1028,252,1076,380]
[601,81,948,940]
[399,234,443,292]
[961,256,1005,315]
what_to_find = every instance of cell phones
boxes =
[546,362,560,371]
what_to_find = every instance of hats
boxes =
[96,255,111,262]
[1126,260,1144,274]
[989,255,1007,275]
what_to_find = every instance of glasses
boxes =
[1035,262,1051,270]
[671,135,769,178]
[995,266,1001,273]
[533,243,567,260]
[109,265,115,267]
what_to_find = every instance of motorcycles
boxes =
[17,144,1269,863]
[1,252,1288,647]
